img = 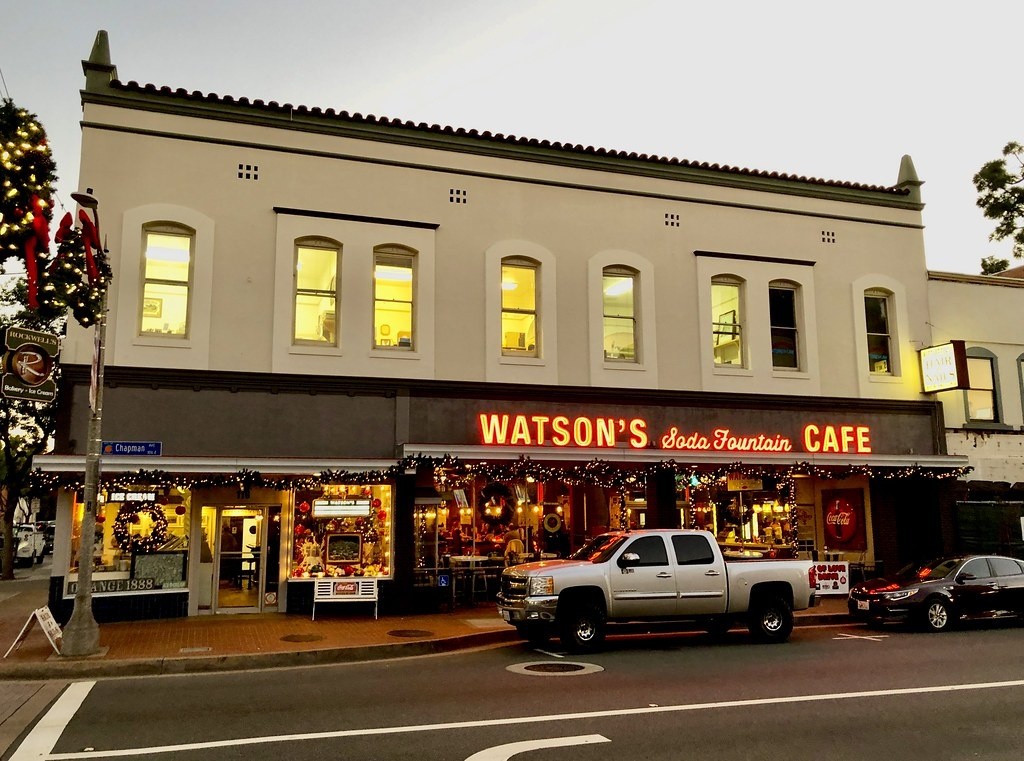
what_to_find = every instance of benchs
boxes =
[312,577,379,621]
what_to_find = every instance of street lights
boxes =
[59,192,109,656]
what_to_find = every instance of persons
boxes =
[220,526,238,586]
[439,520,524,550]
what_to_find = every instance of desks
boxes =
[520,553,556,559]
[452,556,487,596]
[221,551,260,589]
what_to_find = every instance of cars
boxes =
[0,520,56,571]
[849,553,1024,633]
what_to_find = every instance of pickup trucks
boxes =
[496,529,821,653]
